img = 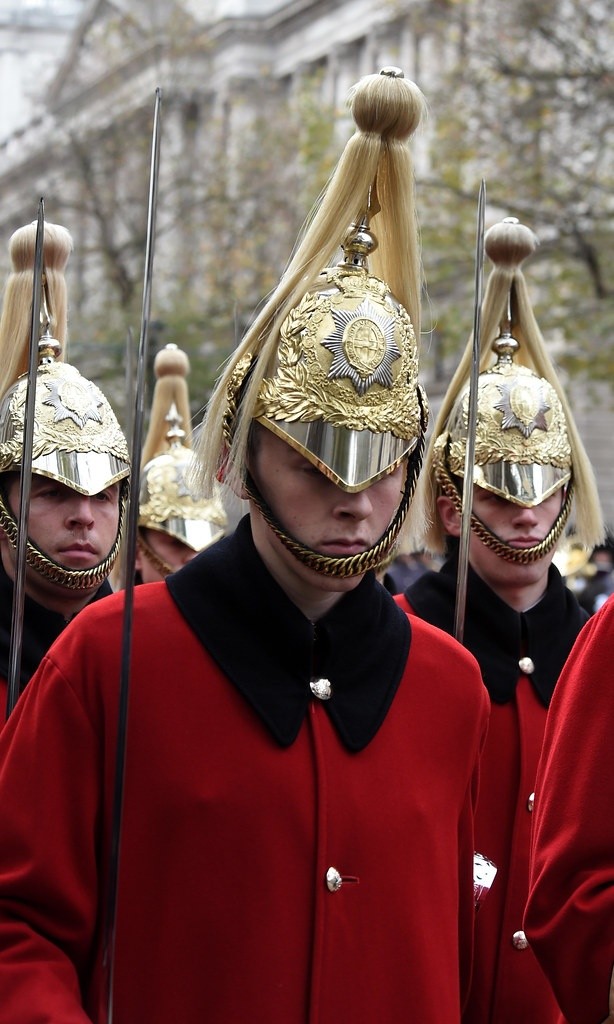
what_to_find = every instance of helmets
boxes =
[430,215,576,510]
[138,404,231,576]
[229,64,434,496]
[0,218,131,495]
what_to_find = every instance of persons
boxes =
[0,218,134,726]
[107,341,231,594]
[0,67,614,1024]
